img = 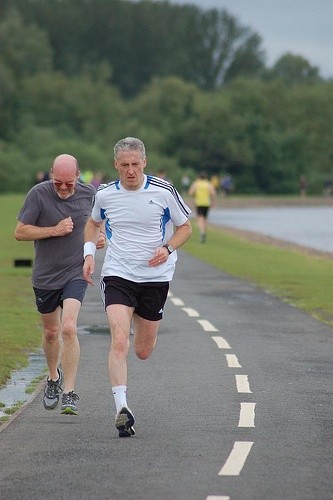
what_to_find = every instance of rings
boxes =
[159,260,161,264]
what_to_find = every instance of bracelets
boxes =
[84,242,96,260]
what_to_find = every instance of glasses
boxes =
[54,182,73,187]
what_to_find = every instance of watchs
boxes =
[162,244,173,255]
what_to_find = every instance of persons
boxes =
[15,154,107,415]
[83,138,191,437]
[34,165,309,243]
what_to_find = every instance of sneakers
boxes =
[42,367,65,411]
[61,391,80,416]
[114,407,135,438]
